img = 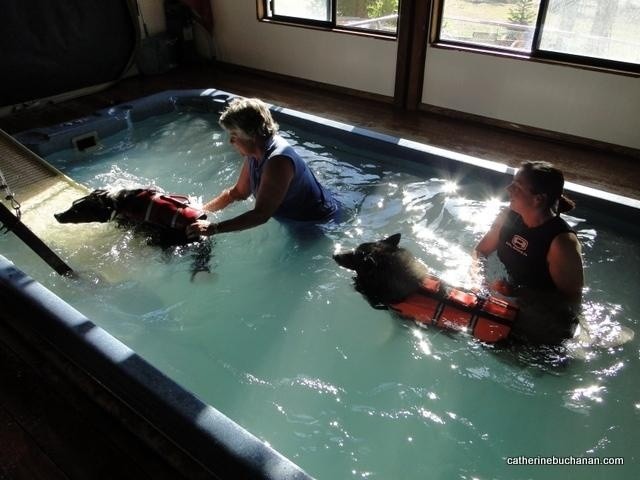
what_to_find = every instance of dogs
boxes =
[54,189,213,282]
[332,232,575,372]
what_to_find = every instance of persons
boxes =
[472,160,583,370]
[185,98,351,239]
